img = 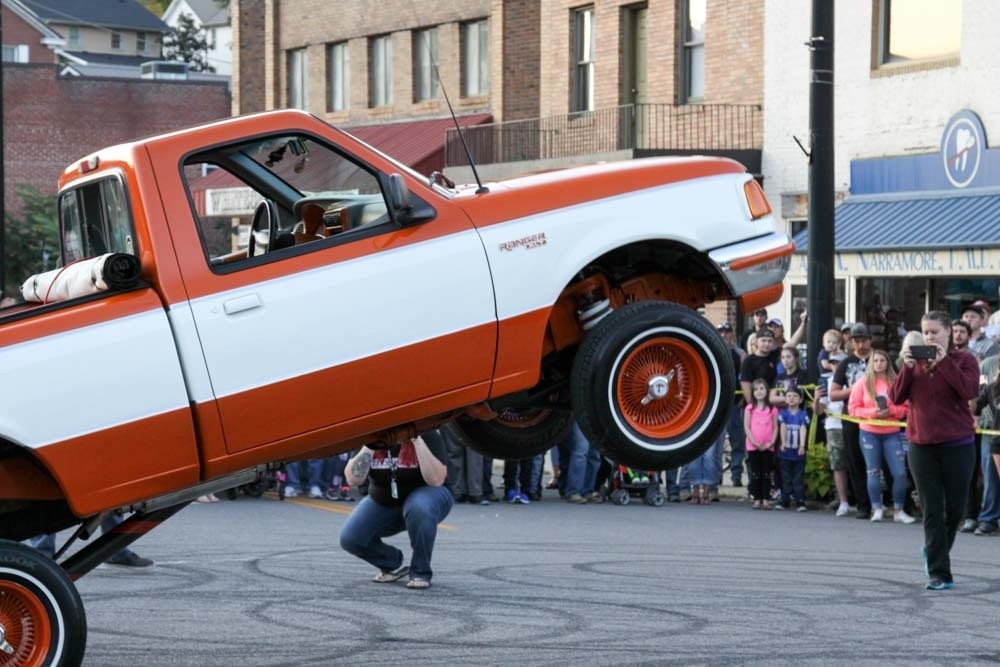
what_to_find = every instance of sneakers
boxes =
[926,577,953,589]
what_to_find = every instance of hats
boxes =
[841,322,875,339]
[953,300,989,336]
[717,308,785,338]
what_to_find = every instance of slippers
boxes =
[373,566,410,583]
[406,576,430,588]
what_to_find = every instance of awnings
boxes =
[783,194,999,277]
[191,112,494,219]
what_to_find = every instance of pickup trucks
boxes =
[0,109,798,667]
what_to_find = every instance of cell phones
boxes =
[910,346,937,359]
[875,396,887,411]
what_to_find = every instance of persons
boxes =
[888,308,980,591]
[277,294,1000,542]
[30,509,151,566]
[339,424,458,591]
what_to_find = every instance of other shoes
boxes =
[975,523,992,535]
[797,503,807,512]
[282,485,358,501]
[668,488,721,505]
[961,519,978,533]
[752,501,761,509]
[454,489,607,505]
[102,550,155,569]
[836,502,849,516]
[894,510,915,524]
[871,509,883,521]
[856,509,868,518]
[775,502,790,509]
[762,502,774,509]
